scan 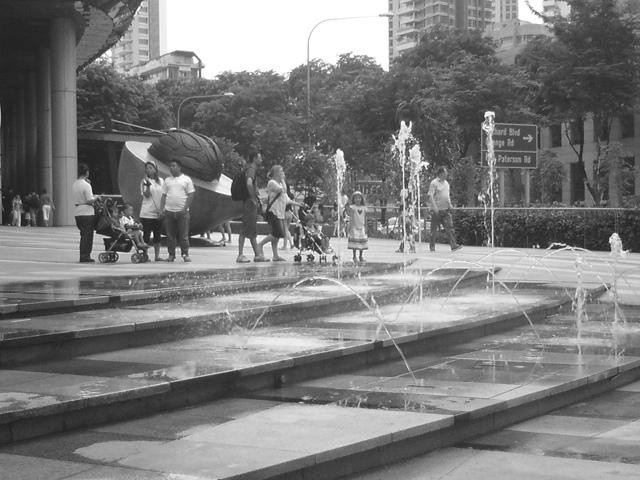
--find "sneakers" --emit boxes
[254,256,270,262]
[183,255,191,262]
[237,255,250,262]
[273,256,286,261]
[168,255,175,261]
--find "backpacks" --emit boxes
[29,193,39,210]
[230,166,252,202]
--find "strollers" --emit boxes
[90,194,151,263]
[286,203,340,264]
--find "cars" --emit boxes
[378,214,429,232]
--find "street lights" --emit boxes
[176,91,236,132]
[305,12,395,157]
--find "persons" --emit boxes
[72,164,102,262]
[139,161,165,261]
[160,159,196,262]
[236,150,270,263]
[428,166,464,252]
[257,165,304,261]
[279,185,338,250]
[200,221,232,243]
[395,189,415,253]
[347,191,369,262]
[2,189,55,227]
[119,203,150,250]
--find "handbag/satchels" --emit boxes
[263,211,275,222]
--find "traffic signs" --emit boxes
[480,122,540,170]
